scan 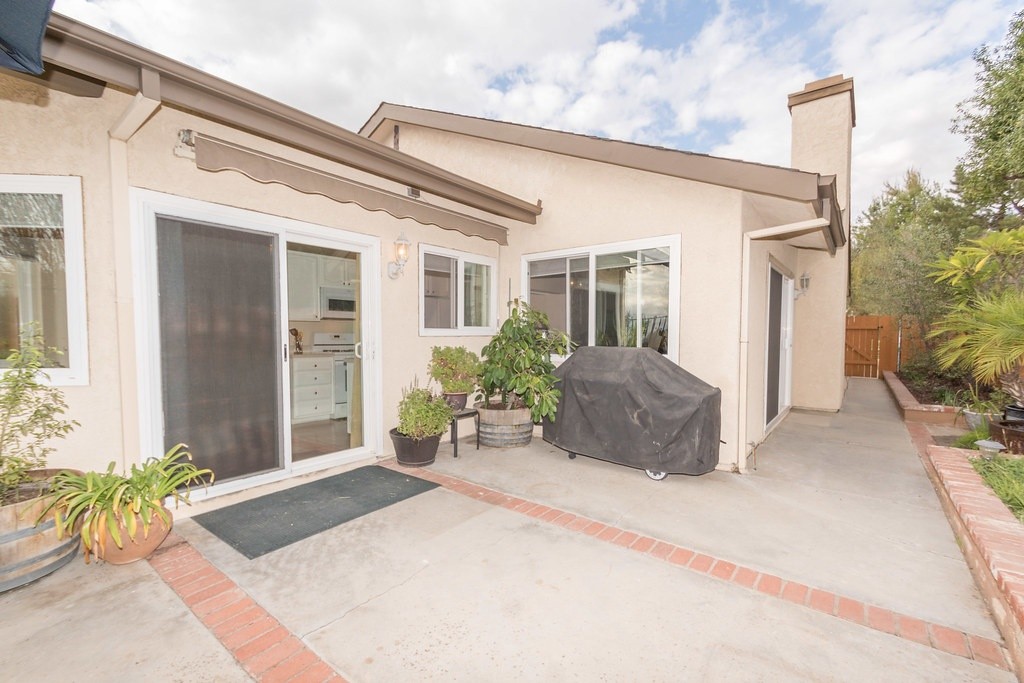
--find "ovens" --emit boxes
[320,286,356,319]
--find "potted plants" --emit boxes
[472,295,580,448]
[924,283,1024,420]
[426,345,482,411]
[15,441,216,566]
[952,377,1005,433]
[0,321,89,594]
[389,373,460,468]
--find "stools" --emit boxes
[437,408,481,458]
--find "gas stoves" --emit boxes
[313,333,355,352]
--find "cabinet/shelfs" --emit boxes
[290,354,335,424]
[319,255,357,290]
[288,251,320,322]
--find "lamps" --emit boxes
[794,270,812,299]
[387,231,412,279]
